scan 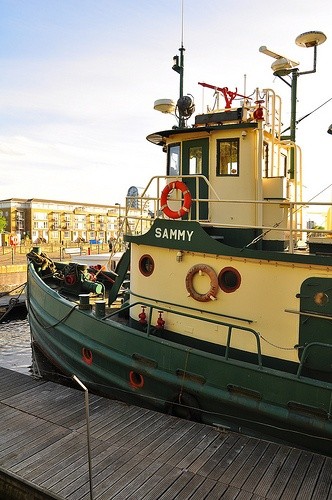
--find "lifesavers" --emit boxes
[185,264,219,303]
[160,181,193,219]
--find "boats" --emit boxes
[23,0,332,459]
